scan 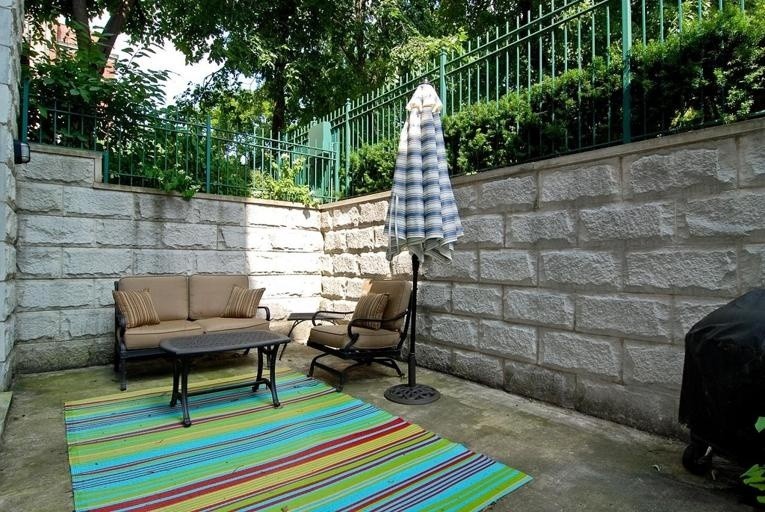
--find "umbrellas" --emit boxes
[378,82,467,354]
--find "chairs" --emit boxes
[306,280,413,393]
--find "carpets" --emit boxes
[63,365,535,512]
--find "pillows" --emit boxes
[351,292,390,331]
[220,284,266,319]
[113,287,161,329]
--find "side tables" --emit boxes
[279,313,346,360]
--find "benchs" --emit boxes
[113,273,270,391]
